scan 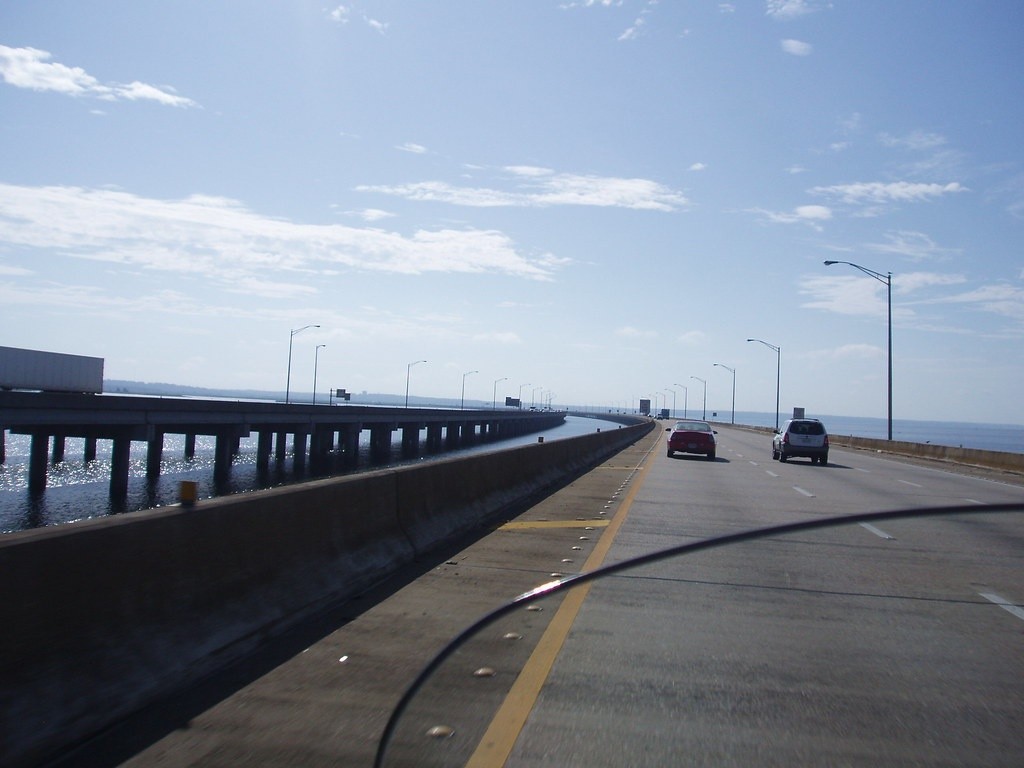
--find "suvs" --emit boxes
[773,418,829,464]
[657,414,663,419]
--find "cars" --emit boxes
[665,420,718,459]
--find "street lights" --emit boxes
[747,339,781,432]
[518,384,531,410]
[674,383,687,419]
[714,363,735,424]
[532,387,554,410]
[286,325,320,405]
[823,260,893,439]
[406,360,427,408]
[494,377,508,410]
[462,371,478,409]
[691,376,706,420]
[664,389,676,418]
[314,345,326,404]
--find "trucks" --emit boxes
[662,409,670,419]
